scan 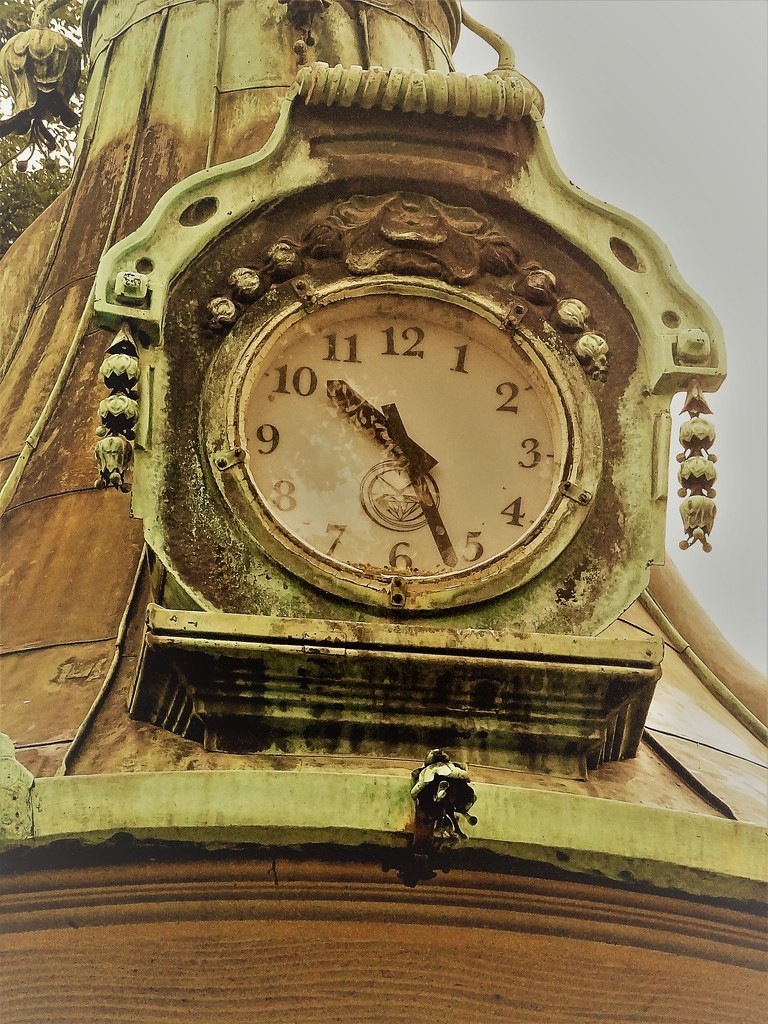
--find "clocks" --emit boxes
[228,274,582,594]
